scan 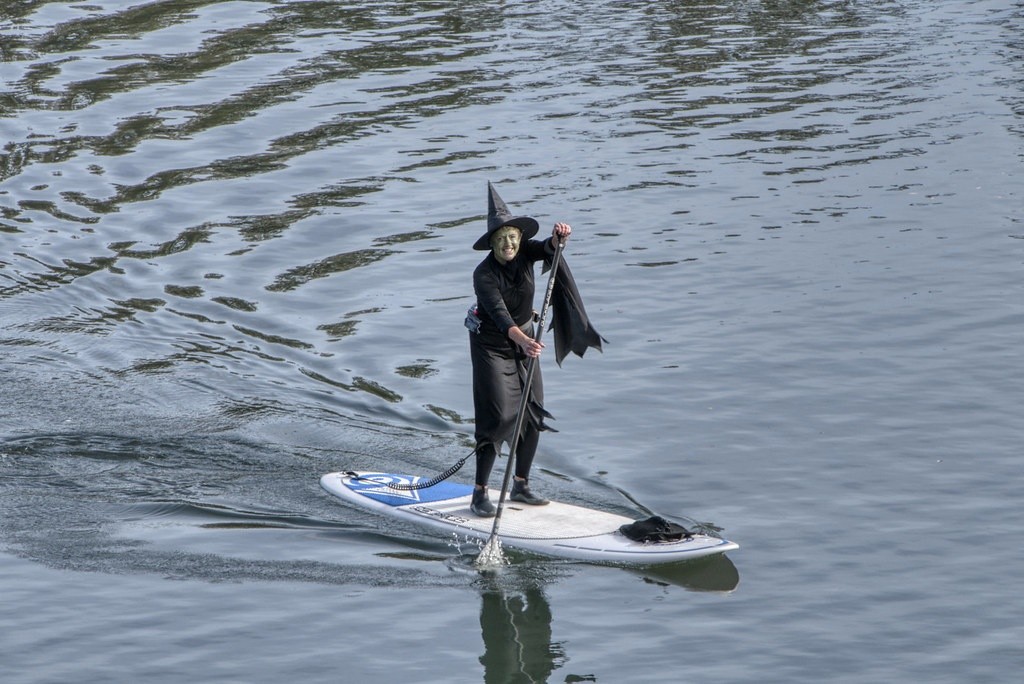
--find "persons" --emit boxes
[469,179,572,518]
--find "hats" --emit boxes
[473,180,539,250]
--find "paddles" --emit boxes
[475,229,569,565]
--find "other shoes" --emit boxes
[510,475,550,505]
[470,485,497,517]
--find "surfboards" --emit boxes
[319,469,741,567]
